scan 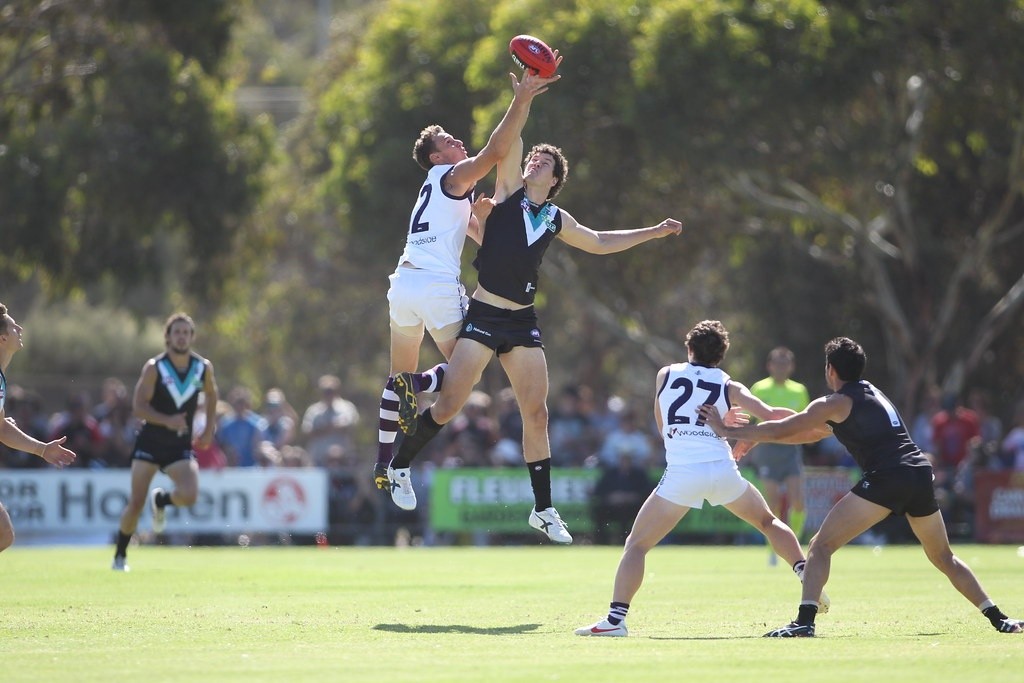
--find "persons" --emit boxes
[387,49,684,544]
[576,319,832,636]
[695,337,1023,636]
[740,348,811,564]
[111,313,219,572]
[374,69,549,495]
[0,376,1024,542]
[0,303,76,551]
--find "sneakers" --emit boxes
[392,372,417,436]
[762,622,815,638]
[373,463,391,496]
[387,457,417,510]
[795,562,831,613]
[113,555,128,571]
[996,616,1024,633]
[528,505,574,545]
[574,617,628,637]
[150,486,167,533]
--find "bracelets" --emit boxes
[41,444,47,458]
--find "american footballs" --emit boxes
[508,33,558,79]
